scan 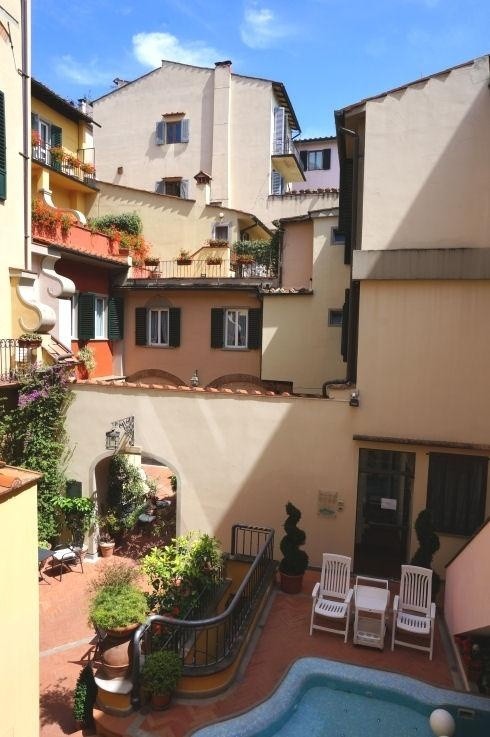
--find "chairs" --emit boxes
[391,564,436,660]
[309,553,353,643]
[53,538,84,582]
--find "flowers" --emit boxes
[141,528,227,651]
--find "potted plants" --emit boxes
[277,502,309,593]
[139,651,184,711]
[18,333,42,349]
[409,508,440,602]
[97,532,115,557]
[75,345,96,380]
[118,239,130,255]
[143,238,257,266]
[86,560,151,637]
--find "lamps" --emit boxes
[189,370,199,387]
[349,389,359,407]
[105,426,120,450]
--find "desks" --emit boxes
[352,576,390,652]
[38,546,57,585]
